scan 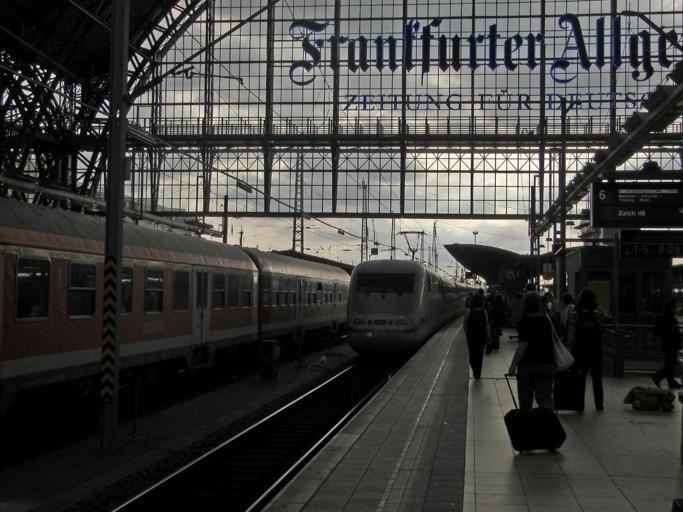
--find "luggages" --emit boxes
[552,362,587,415]
[501,368,569,451]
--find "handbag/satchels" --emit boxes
[622,384,677,412]
[544,309,578,370]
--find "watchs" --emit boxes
[508,367,515,373]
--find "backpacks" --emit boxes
[569,323,603,368]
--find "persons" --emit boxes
[565,287,615,414]
[507,290,558,455]
[462,293,491,379]
[464,283,575,356]
[650,293,683,389]
